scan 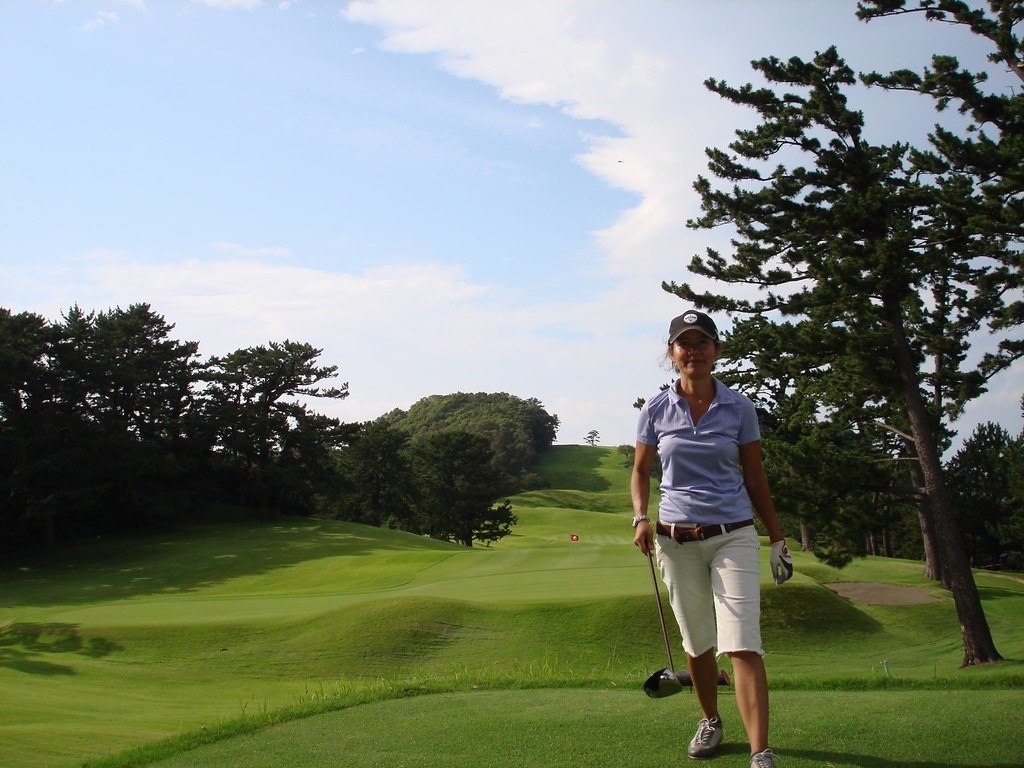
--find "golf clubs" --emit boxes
[643,544,683,698]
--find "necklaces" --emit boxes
[677,389,711,405]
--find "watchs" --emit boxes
[631,514,652,528]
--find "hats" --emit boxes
[668,309,719,347]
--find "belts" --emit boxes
[656,517,755,544]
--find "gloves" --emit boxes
[768,541,794,586]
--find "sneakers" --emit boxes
[688,716,723,760]
[750,748,784,768]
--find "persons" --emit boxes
[631,308,793,768]
